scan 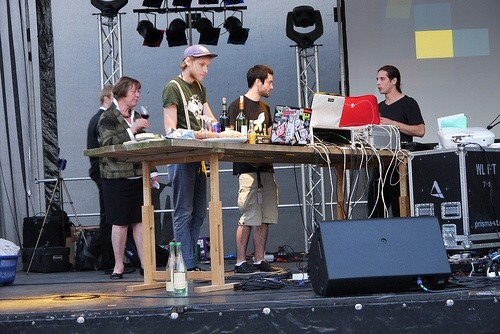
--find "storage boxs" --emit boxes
[408,144,500,251]
[22,247,70,274]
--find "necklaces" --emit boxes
[123,115,131,118]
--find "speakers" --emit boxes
[307,216,451,297]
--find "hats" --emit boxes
[184,44,218,58]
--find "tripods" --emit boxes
[25,166,97,275]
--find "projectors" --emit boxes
[438,127,495,150]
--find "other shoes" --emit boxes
[188,267,206,272]
[105,267,135,274]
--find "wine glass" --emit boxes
[140,105,150,130]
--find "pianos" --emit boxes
[400,141,439,152]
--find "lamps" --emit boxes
[286,5,323,48]
[165,11,188,48]
[136,12,165,47]
[195,10,221,45]
[224,10,249,44]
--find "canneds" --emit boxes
[211,122,221,133]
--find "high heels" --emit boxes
[139,268,144,277]
[111,270,124,279]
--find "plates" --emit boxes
[203,137,245,144]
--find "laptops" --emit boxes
[270,105,313,146]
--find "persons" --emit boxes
[87,85,145,275]
[96,76,158,280]
[360,63,426,222]
[224,63,289,274]
[162,45,218,275]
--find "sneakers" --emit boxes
[234,262,257,275]
[252,260,277,271]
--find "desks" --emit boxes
[84,138,409,294]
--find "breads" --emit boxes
[193,130,244,139]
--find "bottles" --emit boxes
[220,96,230,132]
[235,95,248,138]
[248,120,256,144]
[173,242,188,296]
[165,241,175,297]
